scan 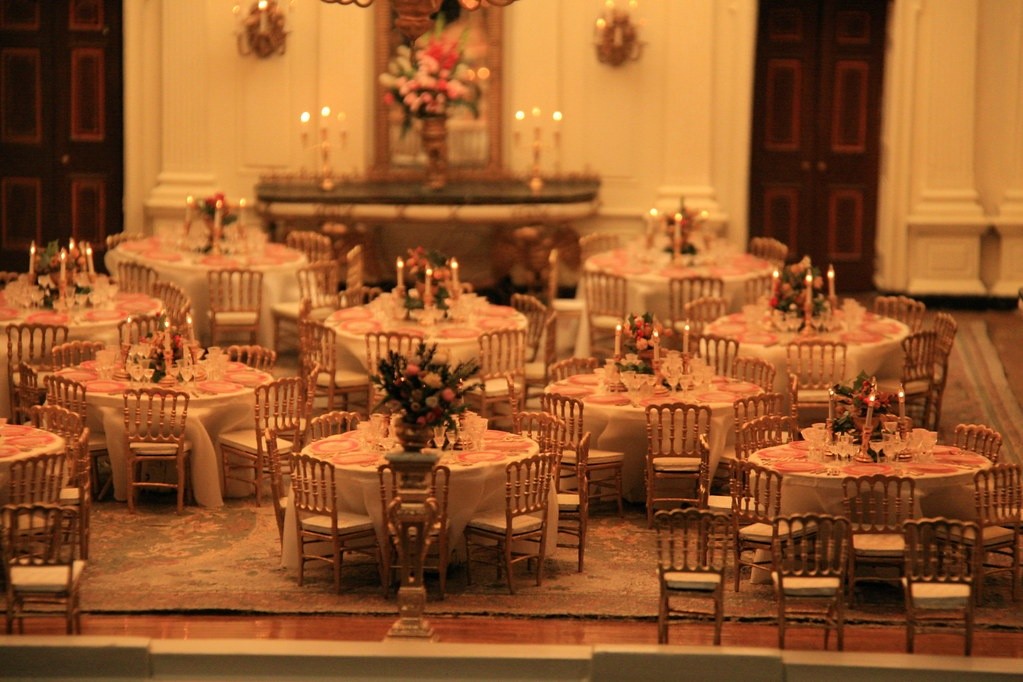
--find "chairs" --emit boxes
[0,231,1023,655]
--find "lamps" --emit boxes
[230,0,290,58]
[593,1,646,67]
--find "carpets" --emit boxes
[0,319,1023,628]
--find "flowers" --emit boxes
[189,192,238,224]
[402,246,463,310]
[379,11,482,140]
[616,313,655,375]
[35,239,91,309]
[368,340,483,449]
[771,265,845,317]
[137,328,183,383]
[666,207,699,236]
[832,368,906,437]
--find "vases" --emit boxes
[422,116,448,189]
[395,417,432,452]
[205,219,225,235]
[639,347,662,362]
[856,416,879,431]
[50,271,73,286]
[415,280,438,295]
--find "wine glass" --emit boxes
[801,422,938,463]
[742,299,867,340]
[371,292,489,332]
[94,345,224,387]
[358,412,488,452]
[4,274,119,314]
[593,354,714,403]
[154,223,269,262]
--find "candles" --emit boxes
[29,197,906,427]
[299,106,563,149]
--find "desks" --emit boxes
[0,424,66,510]
[544,370,767,505]
[40,359,276,509]
[105,237,321,351]
[700,308,910,421]
[749,441,995,584]
[575,249,775,360]
[321,302,528,390]
[282,429,559,563]
[1,281,167,417]
[256,179,601,221]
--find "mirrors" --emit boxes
[372,0,502,181]
